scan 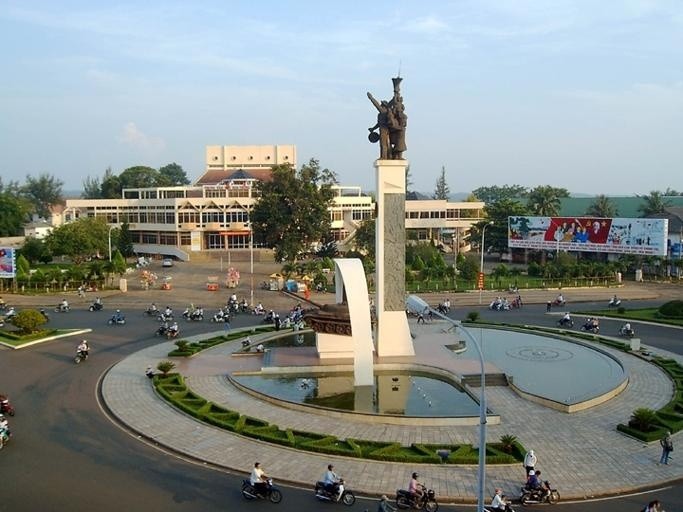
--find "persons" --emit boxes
[316,280,324,292]
[546,301,552,313]
[323,465,345,504]
[389,92,407,160]
[555,294,563,305]
[377,495,396,512]
[112,309,125,323]
[611,295,617,305]
[406,298,450,324]
[5,306,15,315]
[553,220,599,243]
[249,462,268,500]
[485,294,521,312]
[643,501,660,512]
[588,317,598,328]
[365,91,391,160]
[77,339,88,357]
[522,449,537,476]
[0,412,11,432]
[92,296,102,309]
[143,291,304,352]
[557,312,571,326]
[658,431,672,465]
[60,298,68,311]
[489,489,507,510]
[624,320,631,335]
[526,470,548,501]
[407,471,423,509]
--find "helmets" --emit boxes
[495,489,501,494]
[328,464,334,470]
[413,473,418,478]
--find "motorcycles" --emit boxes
[581,318,601,334]
[75,345,92,364]
[315,286,329,296]
[552,299,568,309]
[142,297,304,354]
[393,482,439,510]
[0,393,15,451]
[239,475,283,503]
[616,324,635,337]
[315,477,357,506]
[606,298,622,308]
[0,293,128,330]
[520,481,560,507]
[403,302,453,320]
[487,297,526,312]
[556,316,575,328]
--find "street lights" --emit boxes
[477,220,495,305]
[246,222,255,308]
[108,224,119,263]
[402,292,487,512]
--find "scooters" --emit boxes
[488,502,514,512]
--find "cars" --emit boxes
[162,258,173,267]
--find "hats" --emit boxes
[529,450,534,455]
[529,470,535,476]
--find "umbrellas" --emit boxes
[270,271,283,290]
[300,272,315,288]
